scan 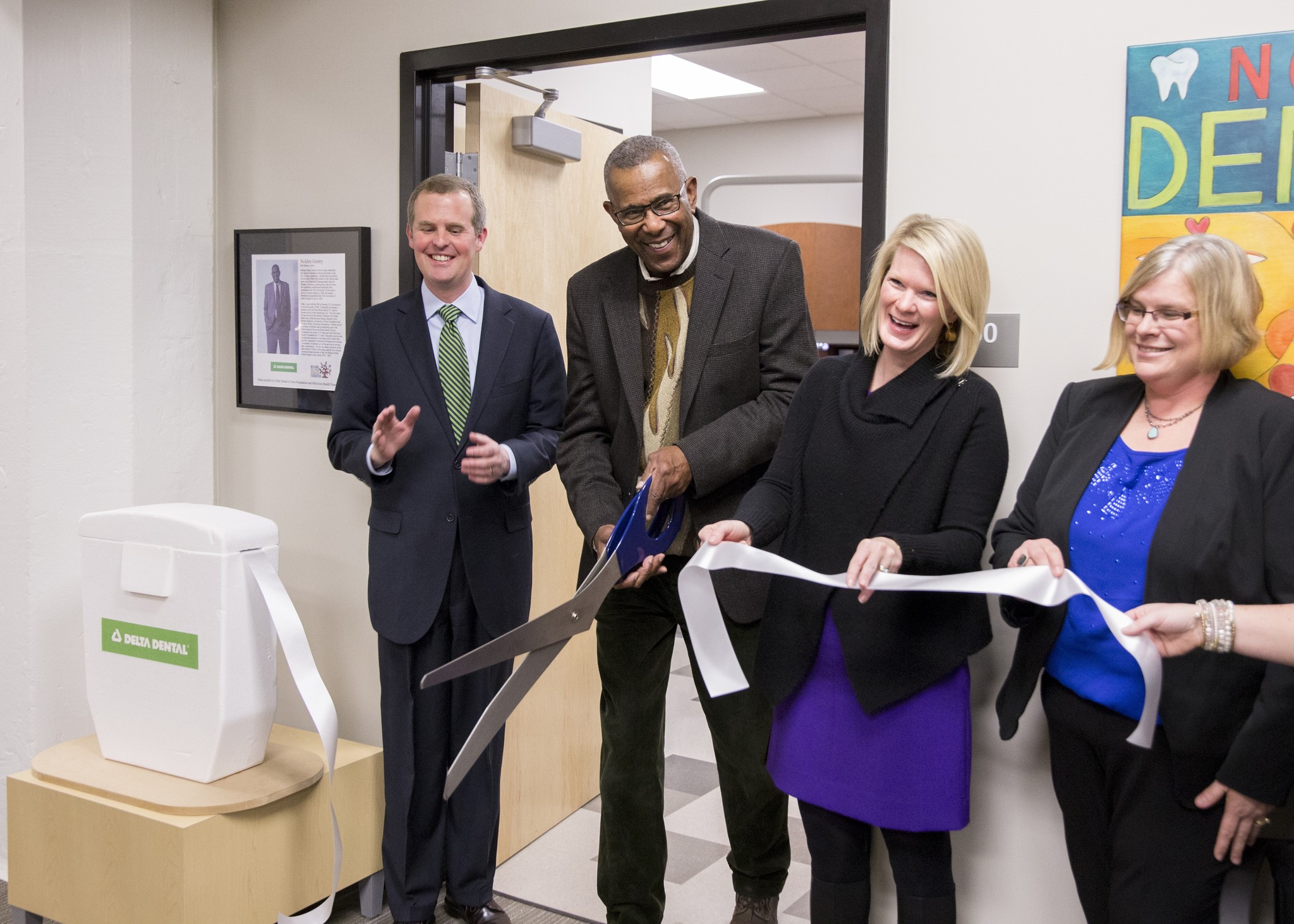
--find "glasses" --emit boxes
[1115,299,1198,327]
[610,178,686,226]
[272,271,279,274]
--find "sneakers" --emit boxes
[729,894,780,924]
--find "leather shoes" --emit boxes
[445,898,511,924]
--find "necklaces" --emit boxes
[1144,404,1202,442]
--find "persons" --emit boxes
[988,235,1294,924]
[1119,602,1294,668]
[698,217,1010,923]
[327,172,567,924]
[563,136,821,924]
[264,265,292,354]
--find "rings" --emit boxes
[491,467,494,476]
[877,564,890,574]
[1017,554,1031,567]
[1254,817,1271,828]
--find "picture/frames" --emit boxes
[233,225,372,414]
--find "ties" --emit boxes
[275,284,281,326]
[437,303,471,450]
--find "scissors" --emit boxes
[421,476,687,800]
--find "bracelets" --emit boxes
[1195,599,1234,654]
